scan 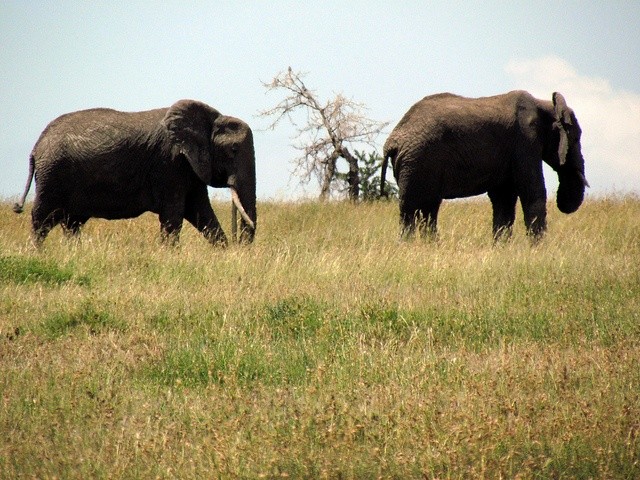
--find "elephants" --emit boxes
[11,99,257,252]
[380,91,591,251]
